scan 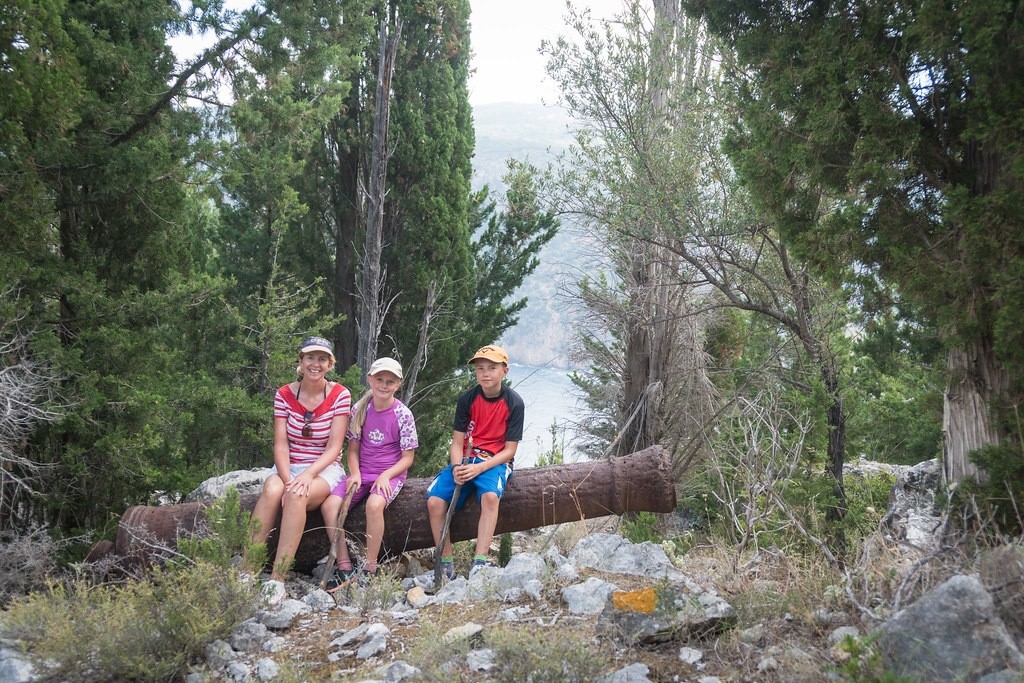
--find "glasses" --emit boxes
[302,411,315,438]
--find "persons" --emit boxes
[235,336,352,607]
[320,357,420,593]
[425,345,526,594]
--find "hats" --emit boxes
[369,357,402,379]
[468,346,508,367]
[302,336,334,356]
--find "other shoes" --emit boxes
[325,570,356,592]
[466,559,485,579]
[427,562,457,594]
[358,569,374,589]
